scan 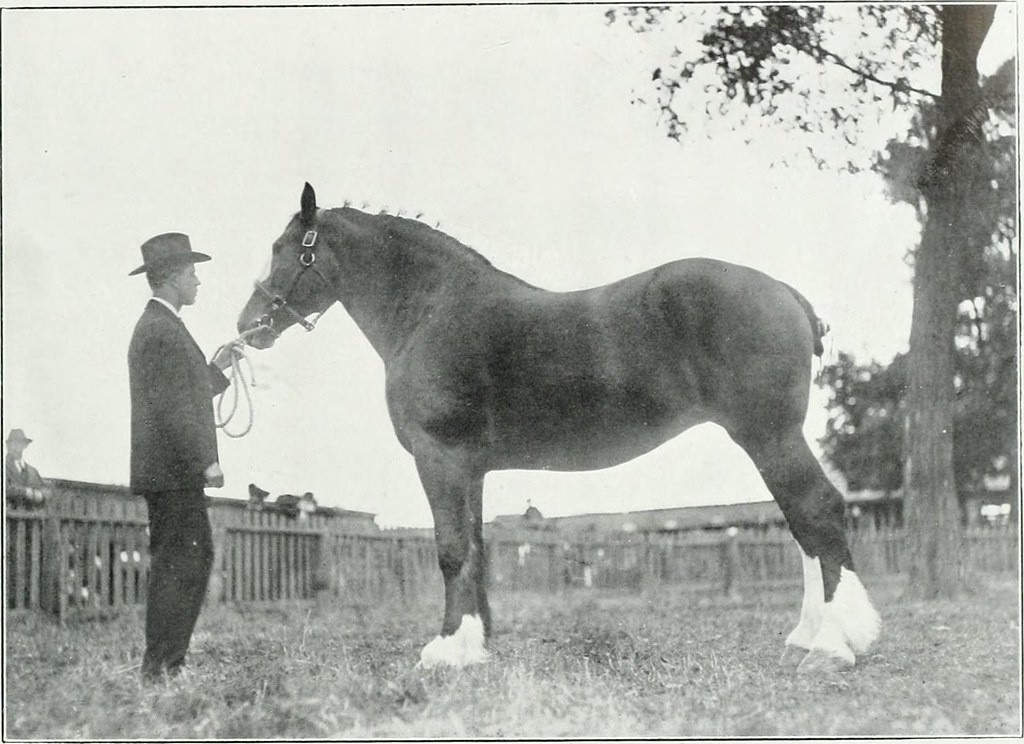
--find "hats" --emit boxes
[129,233,211,276]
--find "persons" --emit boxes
[4,428,50,607]
[128,232,245,689]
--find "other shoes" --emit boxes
[143,667,187,718]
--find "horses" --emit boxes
[236,179,883,677]
